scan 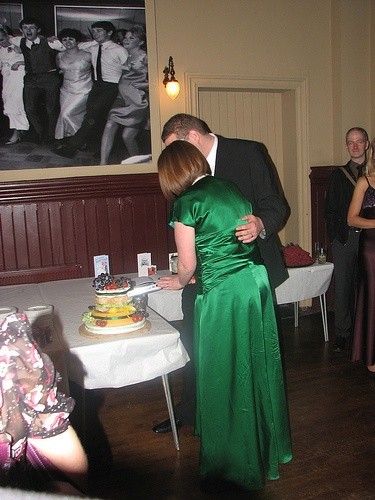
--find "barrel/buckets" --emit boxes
[0,306,16,326]
[23,305,52,324]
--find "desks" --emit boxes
[0,258,335,451]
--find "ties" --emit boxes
[356,165,365,178]
[31,42,35,50]
[96,45,103,85]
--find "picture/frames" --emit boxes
[0,0,160,173]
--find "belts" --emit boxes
[349,227,362,233]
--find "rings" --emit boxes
[167,284,168,287]
[251,233,253,237]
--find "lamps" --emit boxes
[161,57,180,100]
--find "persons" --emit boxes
[0,19,149,165]
[326,127,370,352]
[153,113,291,431]
[347,137,375,371]
[0,314,89,476]
[157,140,293,500]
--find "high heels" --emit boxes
[5,134,22,145]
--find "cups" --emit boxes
[318,247,327,263]
[132,294,147,315]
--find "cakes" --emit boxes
[82,273,143,327]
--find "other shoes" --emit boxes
[51,147,75,159]
[152,415,184,433]
[366,366,375,379]
[332,344,345,352]
